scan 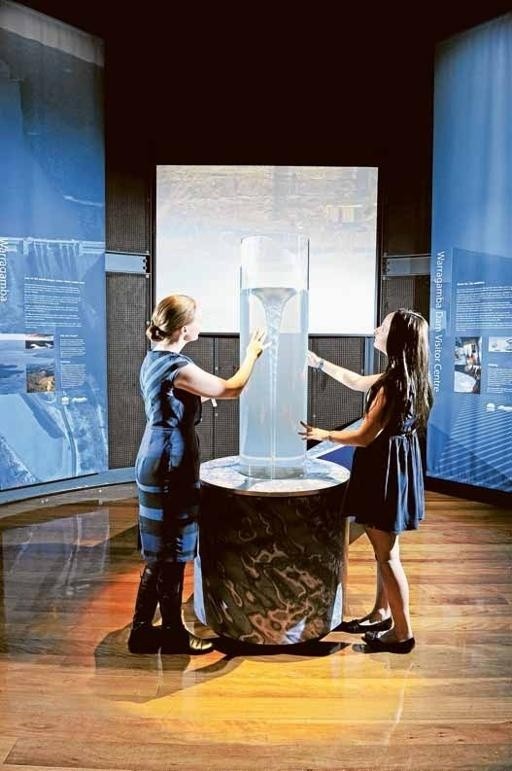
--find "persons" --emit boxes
[128,293,272,654]
[295,307,435,658]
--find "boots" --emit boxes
[128,565,162,653]
[157,563,214,654]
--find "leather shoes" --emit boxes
[345,617,392,633]
[361,632,415,653]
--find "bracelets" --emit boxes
[318,357,325,368]
[327,429,334,443]
